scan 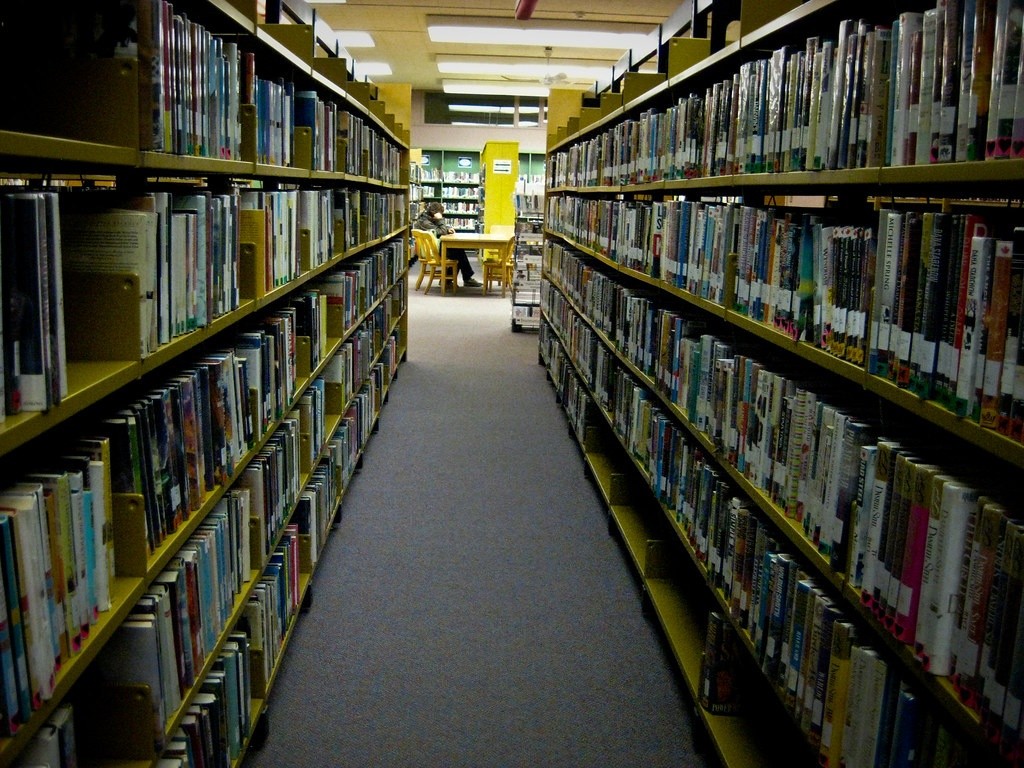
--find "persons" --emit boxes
[416,202,482,290]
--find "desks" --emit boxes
[439,233,511,299]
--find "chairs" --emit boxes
[418,231,459,297]
[482,235,515,299]
[411,228,448,291]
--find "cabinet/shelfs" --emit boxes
[520,0,1024,768]
[0,0,518,768]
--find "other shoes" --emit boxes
[439,281,459,289]
[464,278,481,287]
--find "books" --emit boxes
[512,174,545,327]
[539,0,1024,768]
[409,162,486,259]
[0,237,406,768]
[151,0,401,184]
[0,188,404,425]
